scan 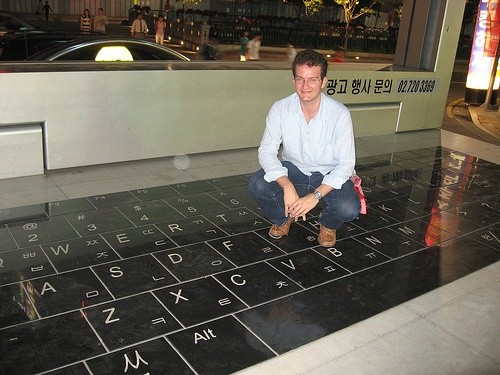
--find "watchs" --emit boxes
[311,189,323,202]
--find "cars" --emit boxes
[23,34,191,63]
[0,13,77,61]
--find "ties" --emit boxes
[139,19,142,31]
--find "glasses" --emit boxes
[295,77,321,84]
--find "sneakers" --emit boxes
[317,223,336,247]
[268,219,293,239]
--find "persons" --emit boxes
[80,9,93,32]
[154,15,166,45]
[92,8,109,33]
[285,41,298,63]
[238,29,261,62]
[248,49,362,247]
[235,251,355,359]
[130,13,149,40]
[35,0,53,25]
